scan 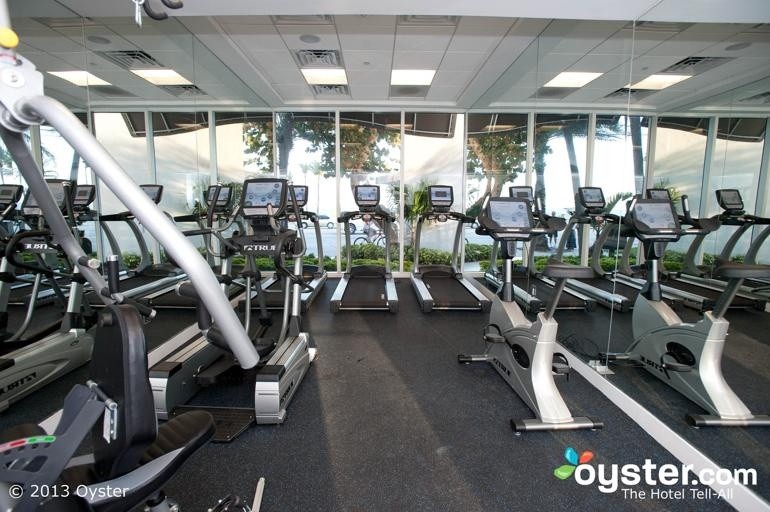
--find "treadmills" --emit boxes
[0,184,766,313]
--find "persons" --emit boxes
[565,226,577,253]
[547,211,559,246]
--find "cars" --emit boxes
[300,213,335,229]
[340,214,369,235]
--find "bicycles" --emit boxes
[352,229,387,249]
